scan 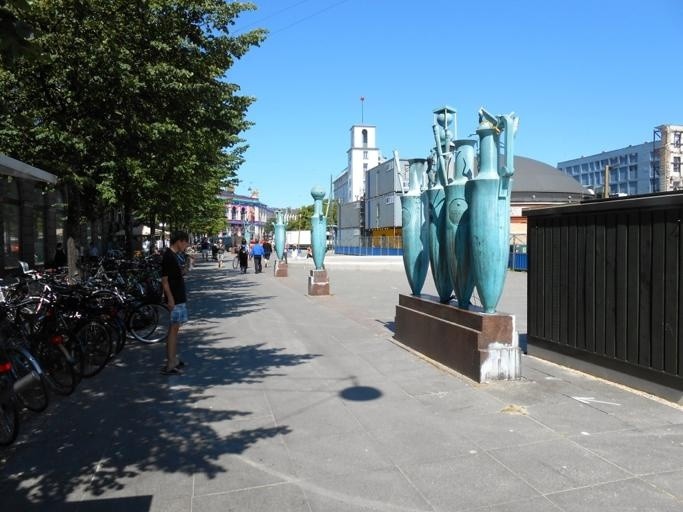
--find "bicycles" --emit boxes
[0,248,169,436]
[231,255,239,269]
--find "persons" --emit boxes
[193,236,298,274]
[159,229,191,374]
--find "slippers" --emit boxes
[175,361,188,369]
[160,369,183,375]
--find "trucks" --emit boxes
[285,230,334,250]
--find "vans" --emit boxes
[201,237,246,252]
